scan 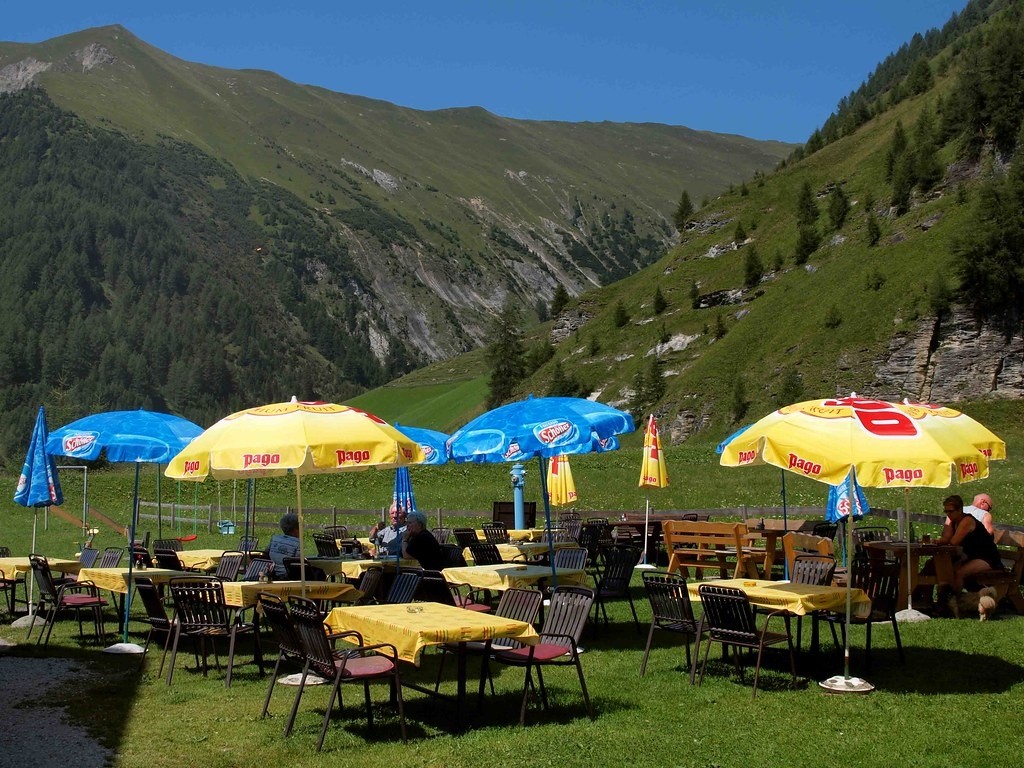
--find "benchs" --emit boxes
[580,520,663,562]
[618,511,711,548]
[660,518,760,580]
[746,518,828,577]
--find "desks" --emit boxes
[0,557,80,614]
[674,578,871,674]
[333,535,381,556]
[861,537,961,616]
[306,557,421,581]
[474,529,565,542]
[202,581,364,662]
[151,548,264,583]
[78,566,205,633]
[440,561,587,628]
[462,541,581,566]
[321,601,540,736]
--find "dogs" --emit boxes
[942,584,996,621]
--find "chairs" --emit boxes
[0,509,906,753]
[968,530,1024,618]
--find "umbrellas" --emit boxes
[634,412,671,569]
[714,390,1024,693]
[446,394,636,655]
[44,406,206,653]
[11,404,65,627]
[164,395,451,607]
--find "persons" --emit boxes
[944,493,994,536]
[369,503,407,593]
[400,511,439,569]
[934,495,1002,618]
[262,513,301,581]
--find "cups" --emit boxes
[923,535,930,544]
[379,547,389,558]
[340,547,346,557]
[362,544,368,552]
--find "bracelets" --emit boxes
[403,541,407,542]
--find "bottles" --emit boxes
[136,554,143,569]
[353,535,359,552]
[374,538,380,553]
[909,522,915,543]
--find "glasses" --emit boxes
[985,501,992,511]
[942,508,958,513]
